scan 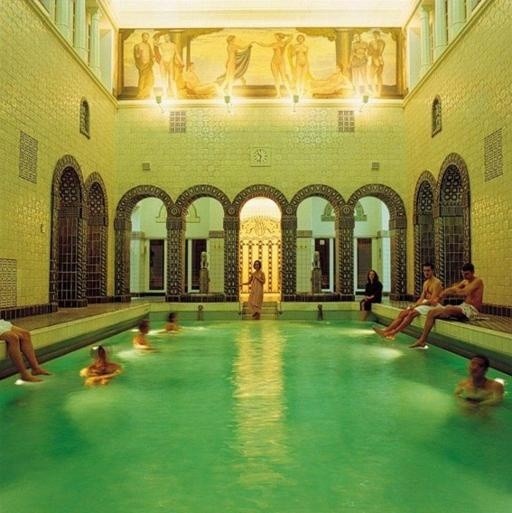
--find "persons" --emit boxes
[235,260,265,320]
[451,354,503,418]
[357,271,384,321]
[132,318,159,352]
[78,345,122,385]
[164,312,183,334]
[133,30,385,100]
[372,263,445,339]
[408,261,485,349]
[0,317,54,384]
